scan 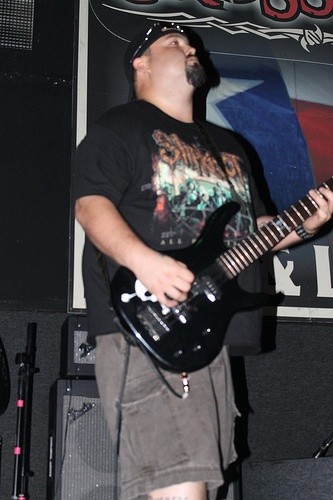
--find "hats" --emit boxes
[126,21,188,67]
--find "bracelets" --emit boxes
[295,225,314,240]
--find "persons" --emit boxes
[72,22,333,500]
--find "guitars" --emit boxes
[111,178,333,374]
